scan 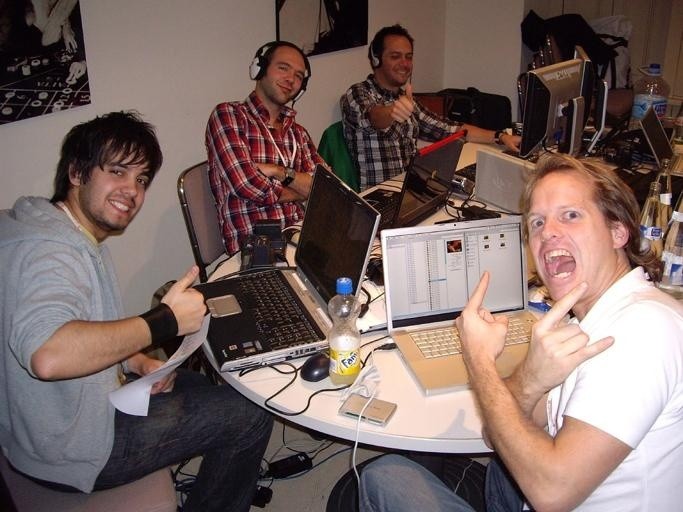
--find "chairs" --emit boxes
[0,450,274,511]
[318,117,360,193]
[175,160,227,282]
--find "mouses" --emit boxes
[300,353,329,381]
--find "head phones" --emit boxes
[369,41,382,69]
[248,38,312,102]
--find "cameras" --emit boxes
[238,219,288,275]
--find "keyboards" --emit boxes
[455,144,520,181]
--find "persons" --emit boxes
[1,107,277,512]
[339,20,522,192]
[201,42,335,255]
[357,150,683,512]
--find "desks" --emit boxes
[194,134,682,512]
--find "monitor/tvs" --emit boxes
[573,46,609,155]
[519,59,585,160]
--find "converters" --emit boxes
[268,452,313,478]
[250,485,272,508]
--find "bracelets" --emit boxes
[493,129,508,146]
[137,302,180,349]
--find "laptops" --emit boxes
[191,163,381,374]
[361,130,468,238]
[639,106,683,177]
[380,215,539,397]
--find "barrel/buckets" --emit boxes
[627,62,670,132]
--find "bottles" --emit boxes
[655,160,672,235]
[328,278,362,387]
[654,191,683,299]
[637,180,663,262]
[627,63,671,143]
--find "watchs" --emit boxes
[279,166,297,188]
